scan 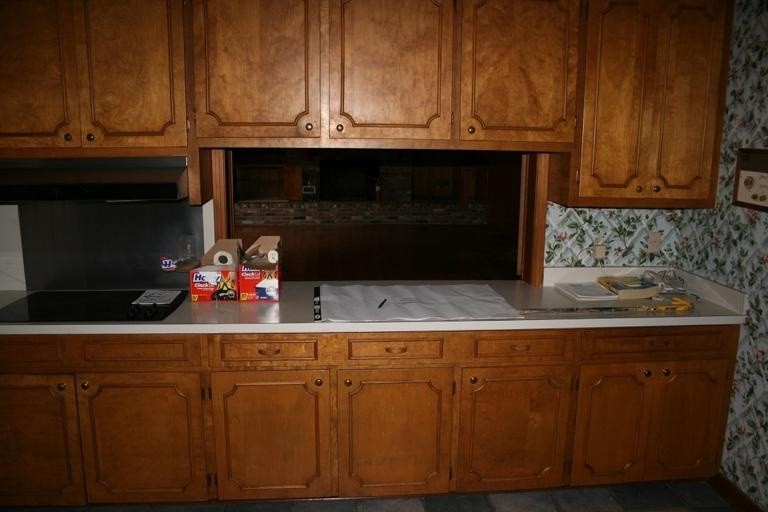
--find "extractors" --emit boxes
[0,160,188,205]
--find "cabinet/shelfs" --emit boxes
[195,1,459,150]
[0,1,195,160]
[211,332,454,511]
[454,332,575,491]
[547,2,734,210]
[1,333,211,509]
[459,2,583,152]
[564,327,742,488]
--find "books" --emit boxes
[555,276,658,302]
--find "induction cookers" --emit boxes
[0,286,183,325]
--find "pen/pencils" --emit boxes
[378,299,386,308]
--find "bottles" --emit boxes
[251,249,278,270]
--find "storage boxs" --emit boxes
[189,233,282,304]
